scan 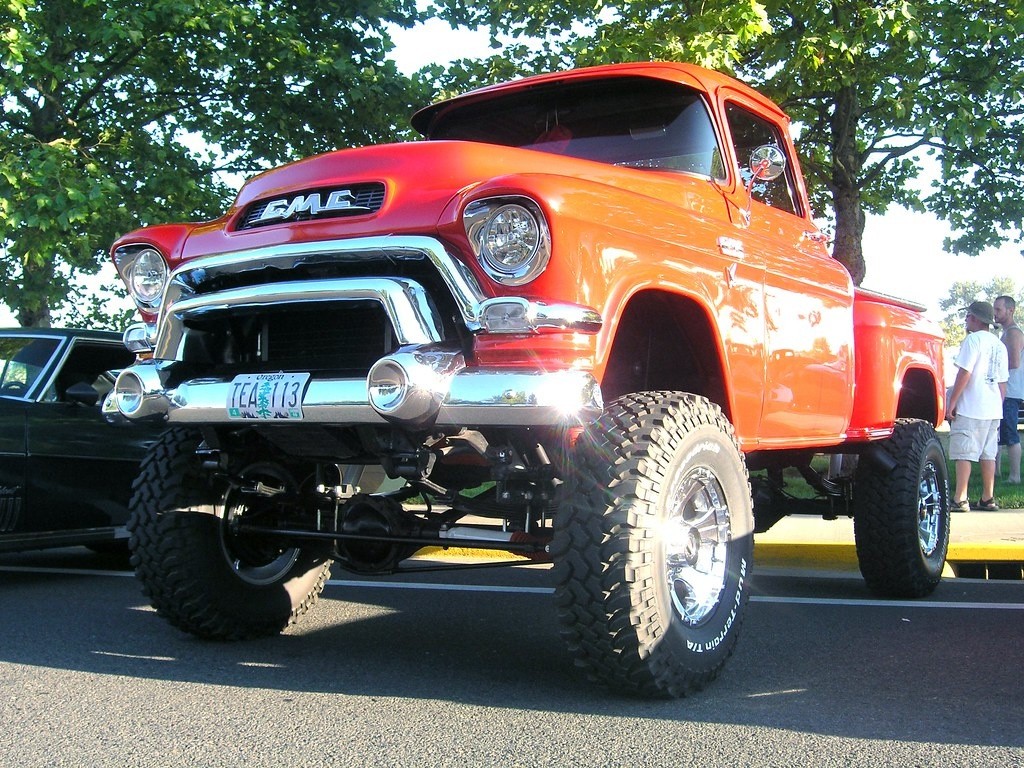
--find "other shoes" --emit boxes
[998,478,1022,487]
[995,475,1002,481]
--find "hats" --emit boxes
[958,302,994,325]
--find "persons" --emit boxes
[943,301,1010,511]
[993,296,1024,485]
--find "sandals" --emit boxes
[971,497,998,511]
[950,500,970,512]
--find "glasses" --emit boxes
[967,312,972,318]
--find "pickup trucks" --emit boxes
[101,61,953,699]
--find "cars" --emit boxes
[0,328,164,567]
